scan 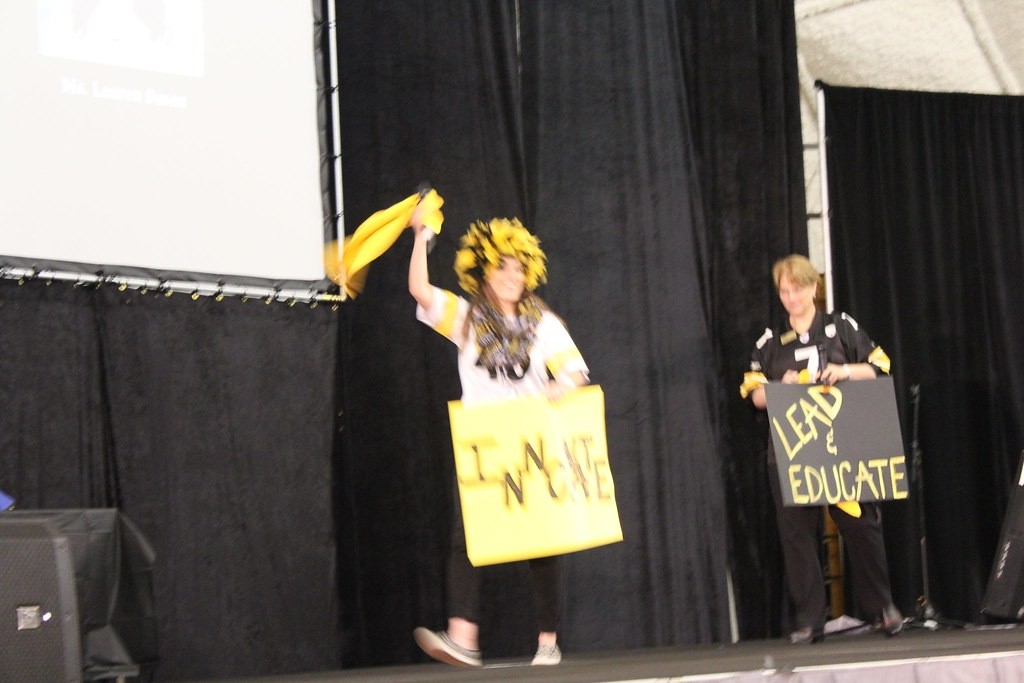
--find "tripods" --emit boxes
[895,385,975,632]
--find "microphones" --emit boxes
[817,344,830,393]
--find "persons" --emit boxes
[741,253,903,642]
[406,174,591,668]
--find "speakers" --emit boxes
[0,512,87,683]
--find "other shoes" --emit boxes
[789,627,818,642]
[881,604,904,638]
[413,628,482,669]
[530,644,561,665]
